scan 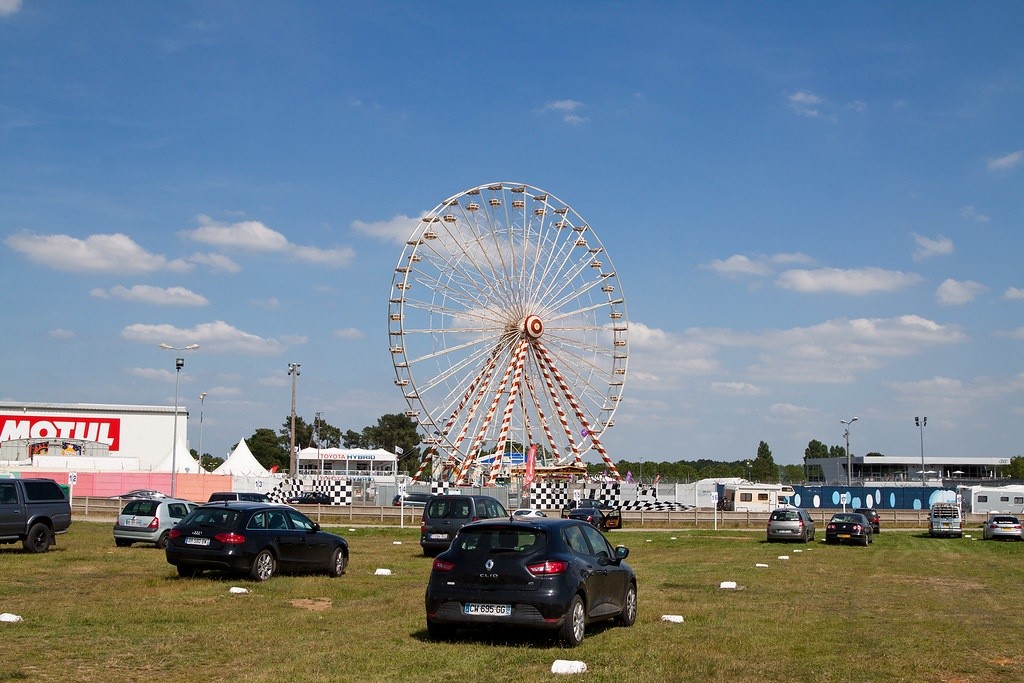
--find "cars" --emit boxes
[108,489,185,501]
[983,515,1024,542]
[561,499,623,532]
[512,509,548,519]
[767,507,815,543]
[114,500,212,550]
[825,514,873,547]
[166,503,350,582]
[287,492,331,505]
[425,519,637,649]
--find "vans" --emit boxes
[420,494,509,557]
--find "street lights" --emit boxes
[915,417,927,485]
[199,393,206,473]
[840,417,858,487]
[159,343,200,500]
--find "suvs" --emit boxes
[209,491,272,515]
[851,508,881,534]
[392,492,445,508]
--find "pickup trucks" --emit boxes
[927,503,963,538]
[0,477,72,554]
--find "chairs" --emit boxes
[175,507,182,517]
[212,514,224,523]
[446,506,464,517]
[269,515,283,529]
[499,532,518,548]
